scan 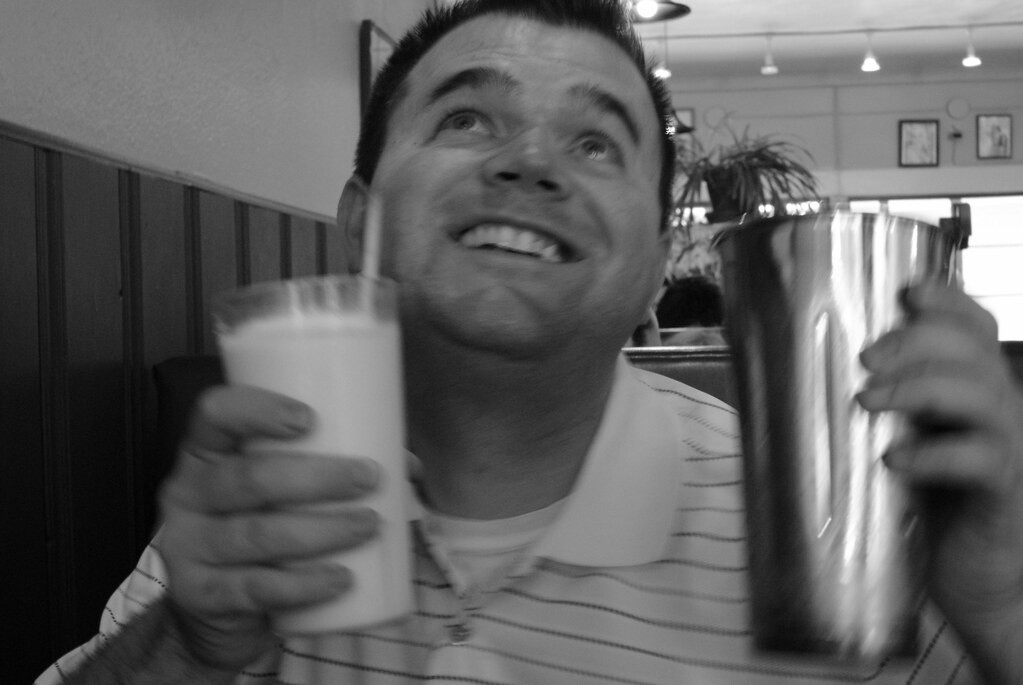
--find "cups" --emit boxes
[210,275,418,639]
[716,203,971,665]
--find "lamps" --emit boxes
[761,35,779,73]
[963,27,983,67]
[628,0,690,23]
[651,22,672,78]
[861,32,880,71]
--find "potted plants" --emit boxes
[670,108,821,224]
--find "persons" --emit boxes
[656,278,725,328]
[31,1,1023,685]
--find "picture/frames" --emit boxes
[976,112,1013,160]
[899,118,939,167]
